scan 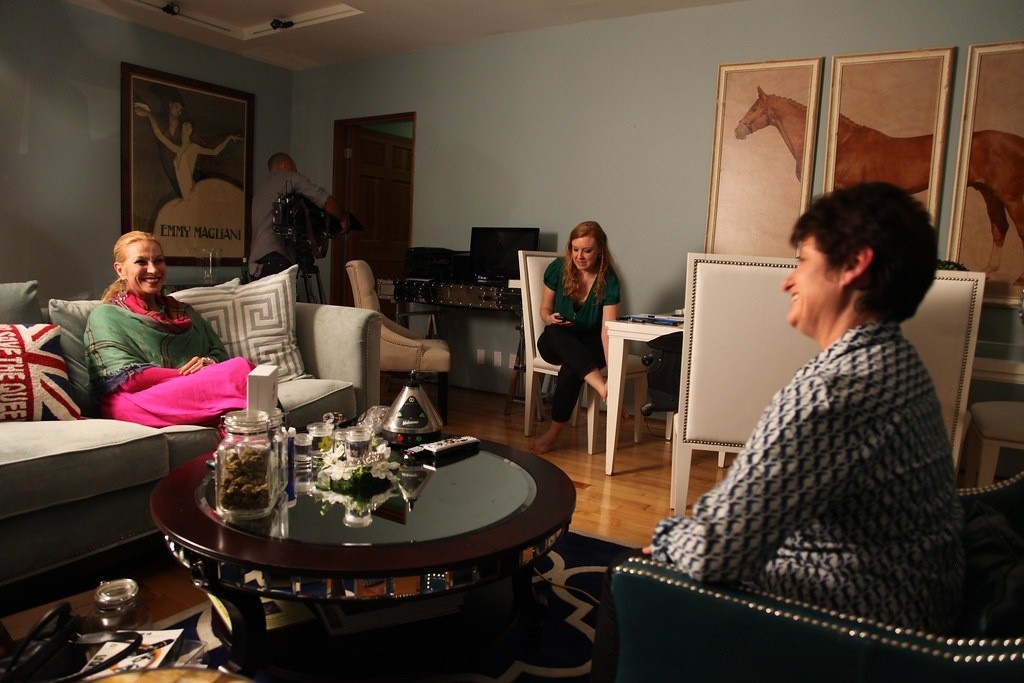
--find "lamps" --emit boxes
[271,19,294,29]
[161,4,180,15]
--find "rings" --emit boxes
[202,362,207,366]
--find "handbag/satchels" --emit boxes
[271,180,331,259]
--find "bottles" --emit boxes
[215,409,371,518]
[241,258,247,281]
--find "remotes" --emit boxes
[405,436,483,458]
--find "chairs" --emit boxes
[346,259,451,381]
[517,246,1024,683]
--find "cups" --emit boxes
[195,248,222,283]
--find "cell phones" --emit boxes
[556,316,570,323]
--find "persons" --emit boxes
[248,153,349,281]
[530,221,629,454]
[591,180,969,683]
[84,231,287,440]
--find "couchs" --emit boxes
[0,302,382,585]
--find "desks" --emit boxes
[378,277,544,421]
[602,319,684,476]
[150,431,575,683]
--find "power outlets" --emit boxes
[493,351,502,366]
[509,353,517,369]
[477,349,485,364]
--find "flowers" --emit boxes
[320,436,400,481]
[312,458,402,515]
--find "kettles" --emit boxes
[382,370,443,445]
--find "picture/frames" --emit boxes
[706,41,1024,308]
[116,62,254,268]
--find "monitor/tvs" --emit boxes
[470,227,540,281]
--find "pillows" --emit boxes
[0,264,314,422]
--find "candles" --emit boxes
[344,427,373,459]
[342,502,372,528]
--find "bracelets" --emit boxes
[207,357,215,364]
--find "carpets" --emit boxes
[145,526,649,683]
